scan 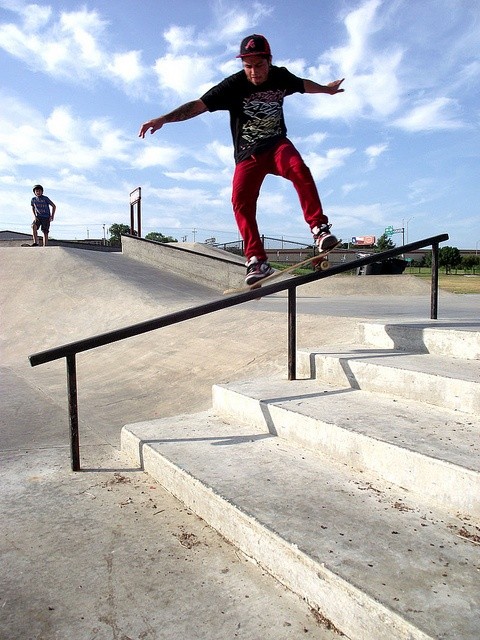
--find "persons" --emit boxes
[139,33,345,285]
[31,185,56,246]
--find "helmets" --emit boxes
[32,184,43,197]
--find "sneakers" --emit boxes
[244,255,276,284]
[312,223,338,252]
[31,243,38,246]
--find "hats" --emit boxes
[235,34,272,59]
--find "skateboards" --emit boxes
[223,239,343,301]
